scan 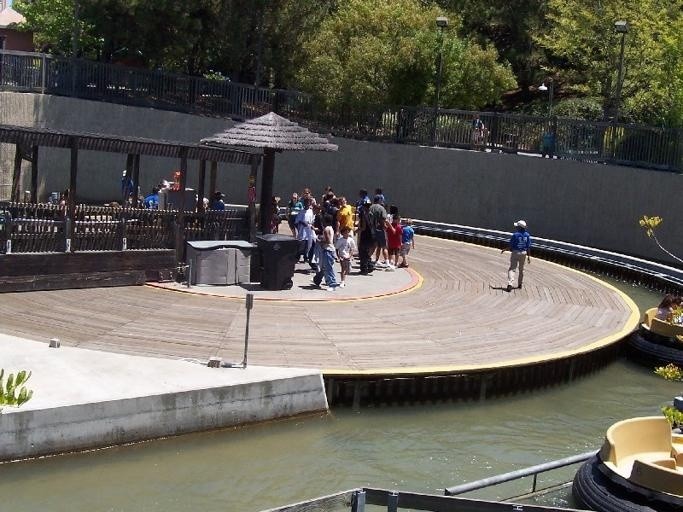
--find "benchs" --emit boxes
[644,306,682,340]
[600,414,683,497]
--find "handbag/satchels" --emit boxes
[367,226,384,248]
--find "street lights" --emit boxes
[610,20,628,160]
[429,17,448,147]
[539,77,552,137]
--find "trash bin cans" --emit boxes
[256,233,299,291]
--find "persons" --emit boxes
[109,170,225,225]
[468,112,485,152]
[541,126,555,160]
[270,185,415,293]
[655,294,683,325]
[498,218,531,293]
[57,194,65,205]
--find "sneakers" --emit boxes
[375,260,409,272]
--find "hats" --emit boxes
[514,220,526,229]
[507,284,522,292]
